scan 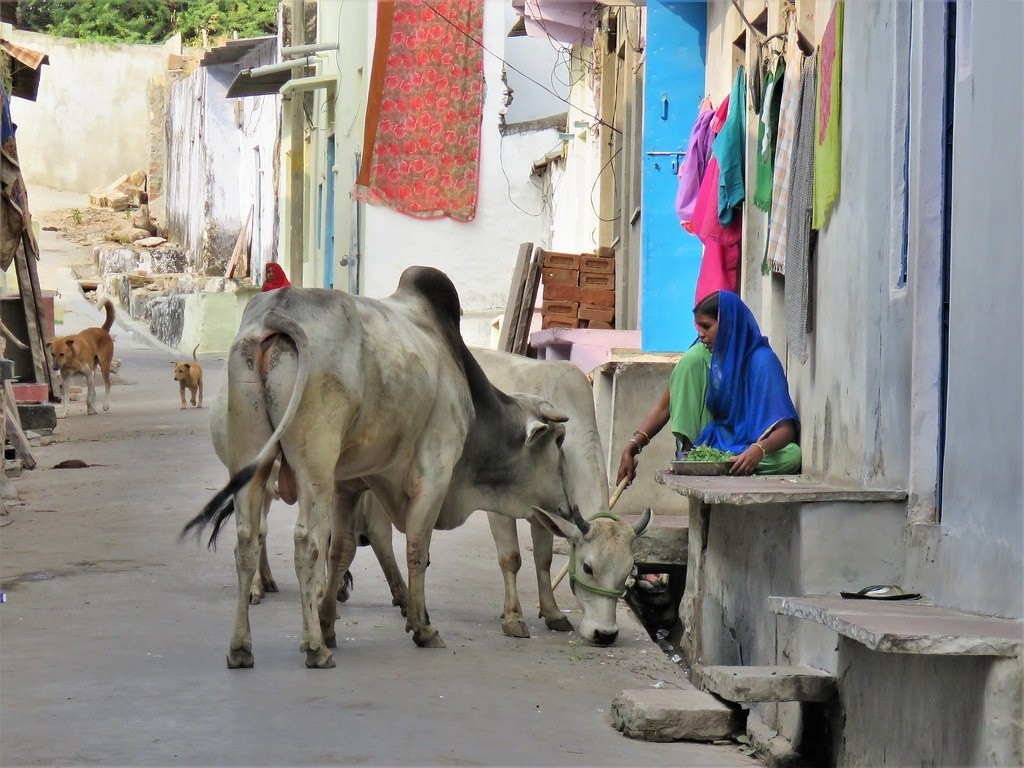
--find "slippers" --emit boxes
[840,584,921,600]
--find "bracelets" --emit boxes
[629,439,641,453]
[633,431,650,446]
[750,443,765,458]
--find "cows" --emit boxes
[176,265,651,672]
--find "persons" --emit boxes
[261,263,291,292]
[617,290,803,489]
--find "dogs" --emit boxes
[170,343,204,410]
[46,296,115,419]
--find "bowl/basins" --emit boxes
[666,459,736,475]
